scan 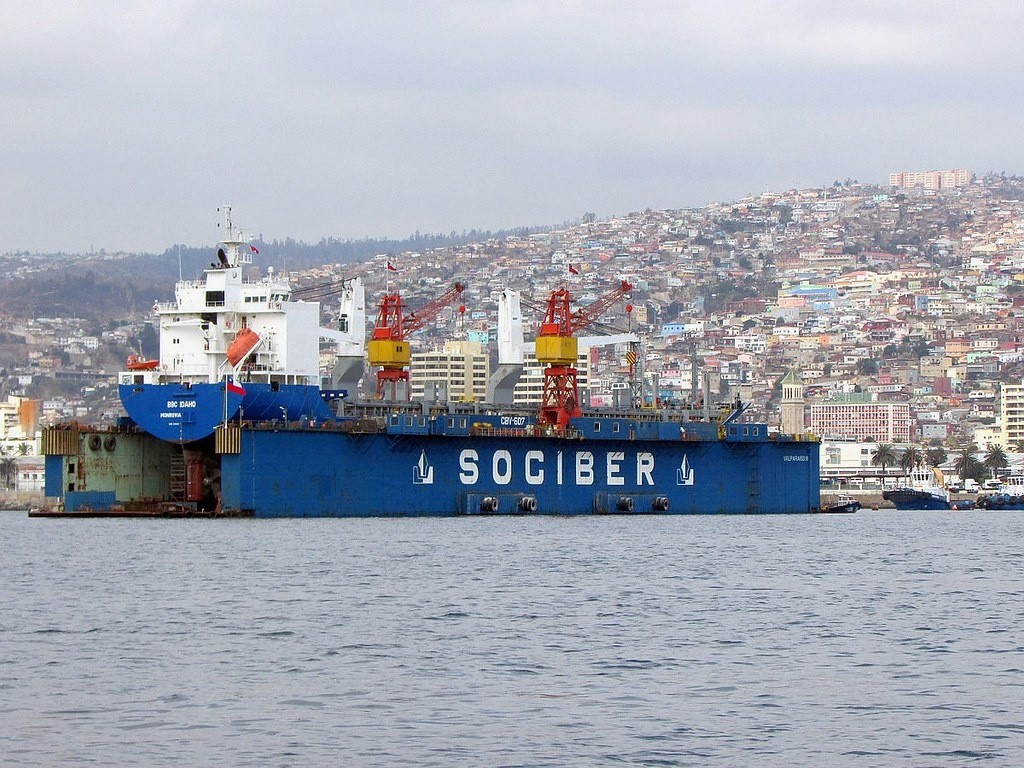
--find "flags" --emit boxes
[226,375,246,395]
[388,262,396,271]
[250,245,258,254]
[569,264,579,274]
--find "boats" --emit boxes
[977,490,1024,512]
[822,490,863,515]
[948,498,976,511]
[884,485,953,510]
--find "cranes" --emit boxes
[366,278,469,404]
[533,278,639,440]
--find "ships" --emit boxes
[32,210,826,520]
